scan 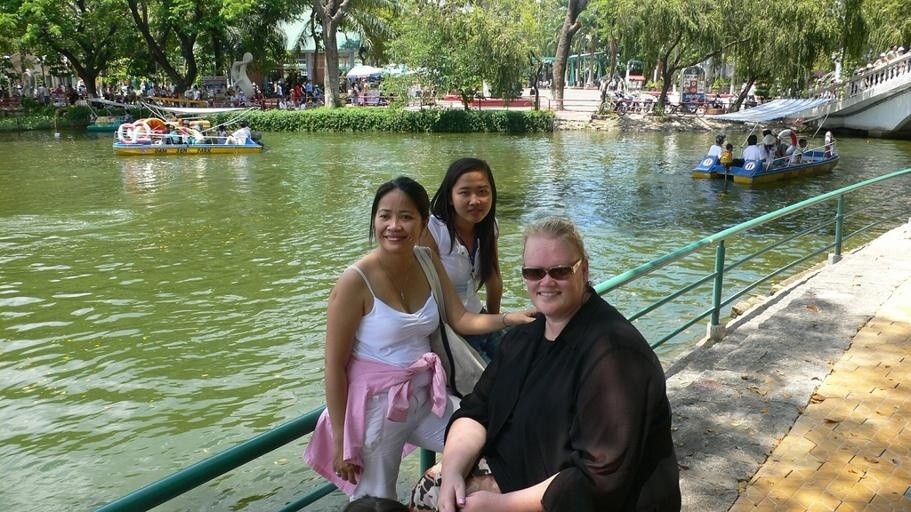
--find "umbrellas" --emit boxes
[346,62,441,81]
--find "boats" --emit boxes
[83,95,137,130]
[691,97,840,184]
[111,97,263,153]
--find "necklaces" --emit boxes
[386,265,415,303]
[452,224,483,314]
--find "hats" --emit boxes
[714,134,725,143]
[763,134,776,145]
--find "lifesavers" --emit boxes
[824,131,833,157]
[133,120,151,143]
[118,124,134,144]
[776,130,797,155]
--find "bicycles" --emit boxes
[603,90,704,118]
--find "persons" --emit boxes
[350,78,440,107]
[708,133,728,161]
[722,142,735,166]
[408,214,684,511]
[714,94,723,109]
[16,78,325,113]
[759,128,808,170]
[428,154,505,318]
[808,39,911,106]
[743,135,768,167]
[303,173,548,503]
[150,120,254,145]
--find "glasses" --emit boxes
[521,259,582,280]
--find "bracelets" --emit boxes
[503,311,511,327]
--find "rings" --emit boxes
[336,471,341,474]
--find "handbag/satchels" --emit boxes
[431,322,487,398]
